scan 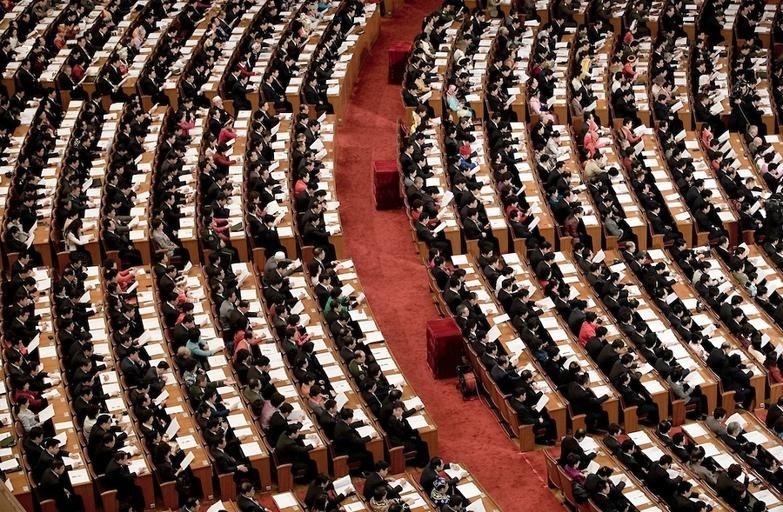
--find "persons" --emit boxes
[0,0,783,511]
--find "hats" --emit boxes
[275,250,286,260]
[211,95,222,103]
